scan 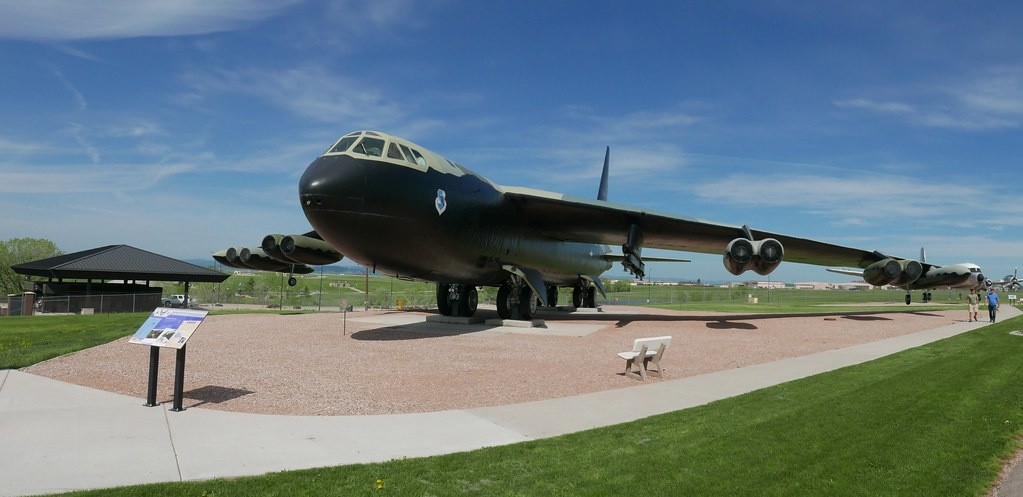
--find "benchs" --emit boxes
[617,335,673,384]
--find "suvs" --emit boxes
[163,295,191,307]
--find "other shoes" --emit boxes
[974,315,978,320]
[990,319,995,322]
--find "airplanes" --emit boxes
[826,246,1023,307]
[209,129,972,320]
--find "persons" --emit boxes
[984,287,1000,323]
[966,289,979,322]
